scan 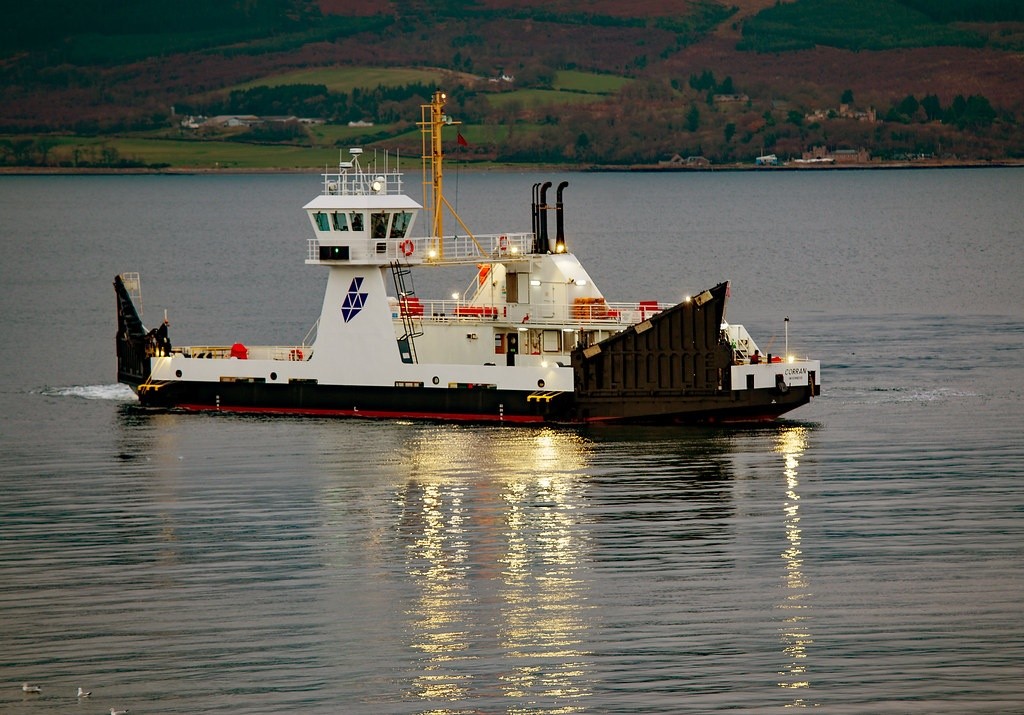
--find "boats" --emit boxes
[111,90,823,428]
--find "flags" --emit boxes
[458,133,468,147]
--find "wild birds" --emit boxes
[77,687,92,698]
[107,707,130,715]
[21,681,43,692]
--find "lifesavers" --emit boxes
[500,237,509,250]
[289,349,303,361]
[402,240,414,255]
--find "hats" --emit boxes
[164,320,169,323]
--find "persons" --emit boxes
[750,349,759,364]
[352,217,361,231]
